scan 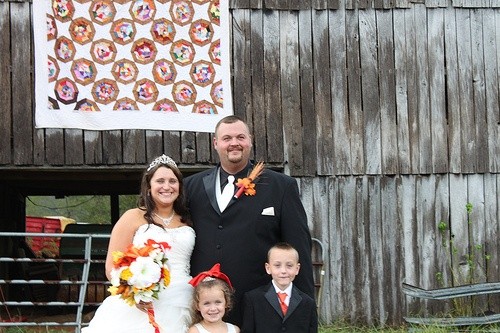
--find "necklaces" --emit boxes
[147,203,181,233]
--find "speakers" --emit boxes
[59,223,116,281]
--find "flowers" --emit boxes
[106,239,172,333]
[234,159,265,198]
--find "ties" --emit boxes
[222,176,235,210]
[278,292,288,316]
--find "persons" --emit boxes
[242,243,319,333]
[80,155,196,333]
[175,115,319,333]
[186,263,240,333]
